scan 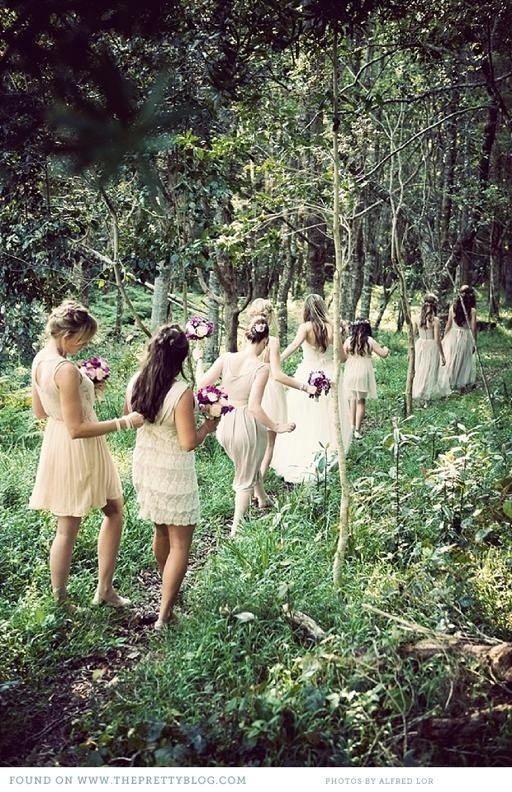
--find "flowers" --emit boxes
[182,315,217,342]
[193,384,236,420]
[305,369,331,400]
[75,354,114,386]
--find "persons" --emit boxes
[269,294,353,484]
[411,294,446,408]
[31,300,145,609]
[192,319,296,537]
[343,318,389,439]
[243,298,317,497]
[123,326,221,631]
[441,285,477,395]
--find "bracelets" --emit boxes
[203,423,211,433]
[300,384,307,391]
[114,416,135,432]
[195,357,202,367]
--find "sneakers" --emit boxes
[423,402,430,409]
[259,495,274,507]
[93,585,133,606]
[355,431,363,439]
